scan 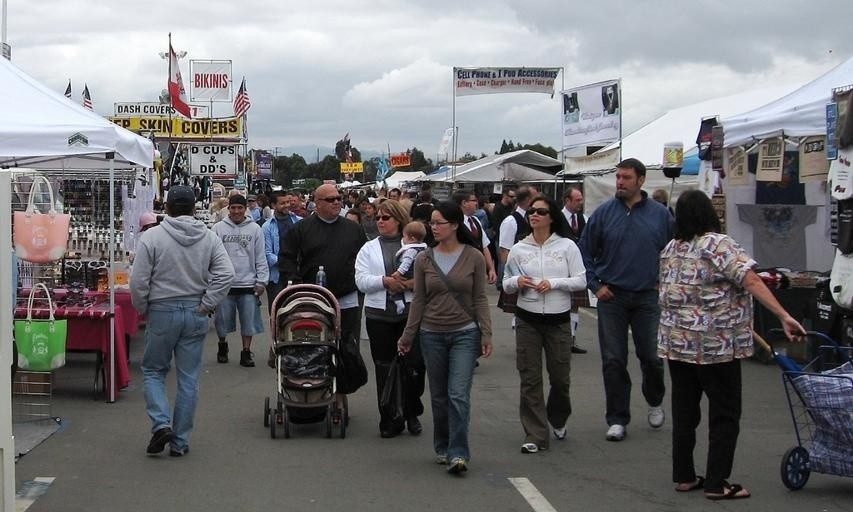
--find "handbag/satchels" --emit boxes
[15,320,67,371]
[13,211,71,263]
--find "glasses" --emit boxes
[65,260,82,272]
[88,260,106,270]
[319,195,549,226]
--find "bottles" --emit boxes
[286,280,292,286]
[316,266,327,288]
[98,268,108,292]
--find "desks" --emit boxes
[12,304,132,400]
[18,286,145,363]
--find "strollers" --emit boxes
[263,282,349,440]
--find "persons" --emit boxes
[577,157,677,441]
[658,189,806,500]
[138,209,164,233]
[128,186,235,459]
[652,188,675,216]
[397,202,493,474]
[353,200,425,438]
[212,184,587,366]
[501,195,589,452]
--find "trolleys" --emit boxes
[767,326,852,491]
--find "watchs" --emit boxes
[67,225,123,261]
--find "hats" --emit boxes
[138,186,257,231]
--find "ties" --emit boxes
[468,218,479,238]
[572,215,577,234]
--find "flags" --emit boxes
[63,84,71,98]
[83,85,94,113]
[232,82,251,120]
[169,45,192,120]
